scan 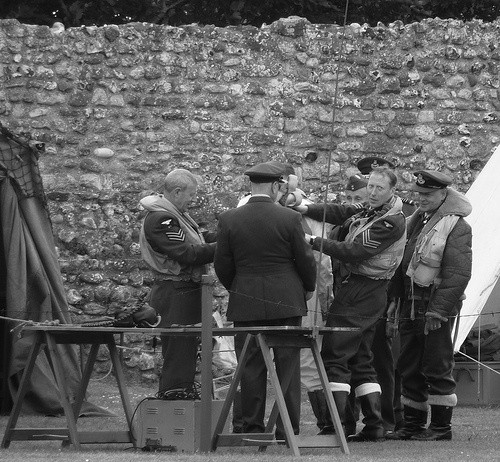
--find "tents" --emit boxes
[0,121,114,417]
[445,143,499,356]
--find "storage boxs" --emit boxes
[452,362,500,406]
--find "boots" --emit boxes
[347,383,385,442]
[308,390,326,430]
[411,394,458,441]
[384,395,429,440]
[315,381,351,436]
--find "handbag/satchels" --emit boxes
[113,306,161,329]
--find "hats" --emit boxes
[357,156,395,178]
[410,170,452,194]
[345,174,367,192]
[244,160,285,184]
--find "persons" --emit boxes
[141,169,217,399]
[215,158,473,442]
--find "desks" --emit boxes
[1,323,361,456]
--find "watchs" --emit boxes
[309,235,317,245]
